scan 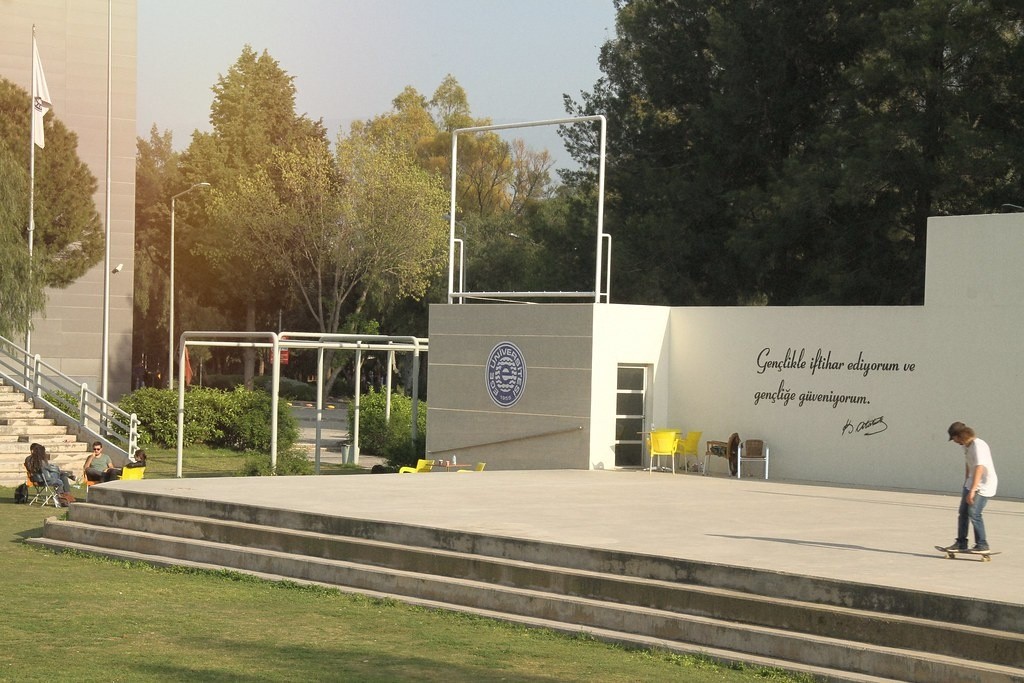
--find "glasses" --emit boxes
[92,448,102,450]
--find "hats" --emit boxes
[948,422,965,442]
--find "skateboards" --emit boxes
[934,545,1003,562]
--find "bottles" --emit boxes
[452,453,456,466]
[650,423,655,432]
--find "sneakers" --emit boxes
[968,543,990,553]
[946,539,968,551]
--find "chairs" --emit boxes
[737,439,770,480]
[645,430,679,474]
[674,430,703,473]
[701,433,741,476]
[23,463,51,506]
[116,465,146,481]
[456,462,487,473]
[399,458,435,474]
[28,464,65,508]
[83,466,101,498]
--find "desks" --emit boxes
[636,431,684,471]
[425,463,472,472]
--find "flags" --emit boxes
[31,33,50,152]
[185,347,193,389]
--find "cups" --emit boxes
[446,461,450,466]
[438,460,443,466]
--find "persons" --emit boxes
[25,443,72,499]
[84,442,115,483]
[947,422,998,554]
[106,450,147,480]
[30,446,76,503]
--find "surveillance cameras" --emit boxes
[114,263,123,274]
